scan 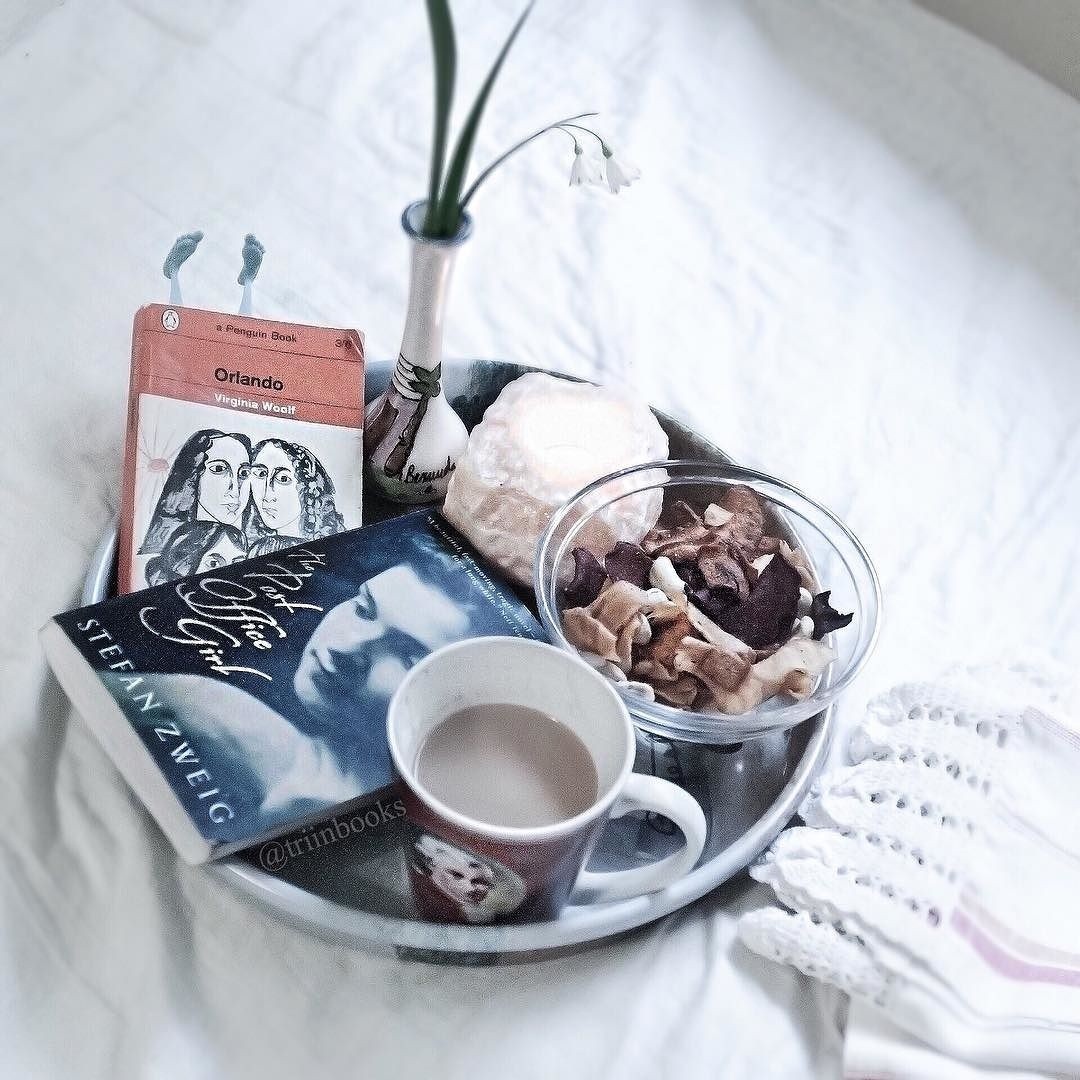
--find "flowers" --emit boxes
[365,0,638,240]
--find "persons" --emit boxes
[82,533,514,856]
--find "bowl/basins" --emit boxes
[532,458,884,746]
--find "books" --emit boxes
[118,302,367,622]
[39,508,543,862]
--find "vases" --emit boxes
[366,202,473,506]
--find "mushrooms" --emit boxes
[562,488,853,718]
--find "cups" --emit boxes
[385,634,709,927]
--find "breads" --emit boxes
[442,371,669,591]
